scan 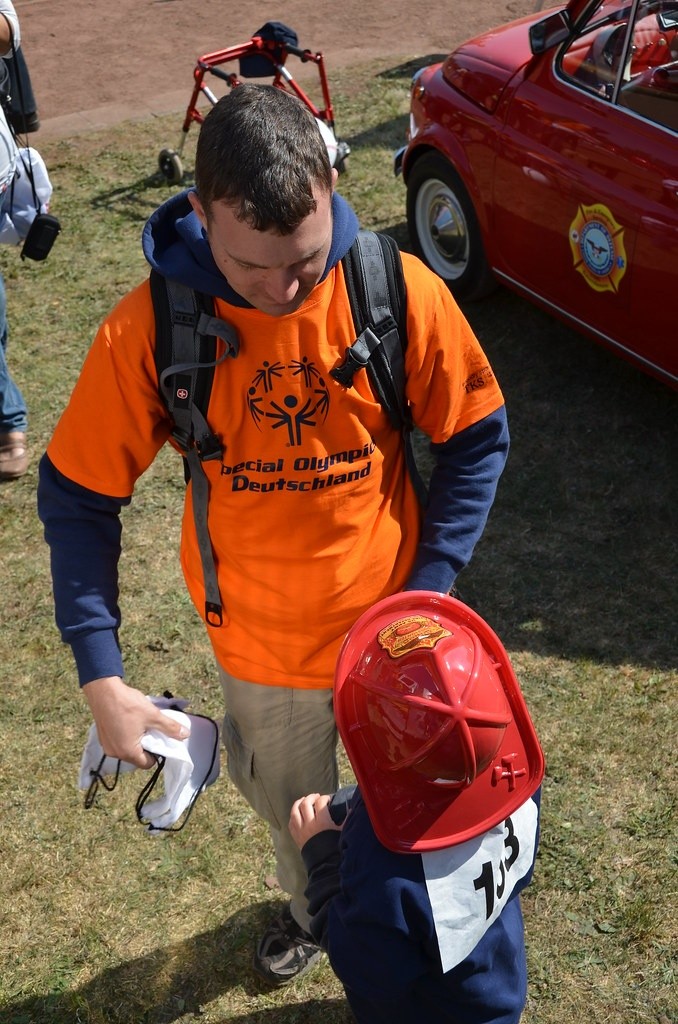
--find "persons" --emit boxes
[287,590,544,1024]
[37,90,510,986]
[0,0,28,480]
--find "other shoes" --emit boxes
[0,432,28,478]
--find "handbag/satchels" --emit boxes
[0,147,61,261]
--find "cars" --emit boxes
[393,0,678,387]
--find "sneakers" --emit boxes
[252,906,324,985]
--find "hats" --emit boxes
[239,23,298,78]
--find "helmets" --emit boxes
[334,591,545,852]
